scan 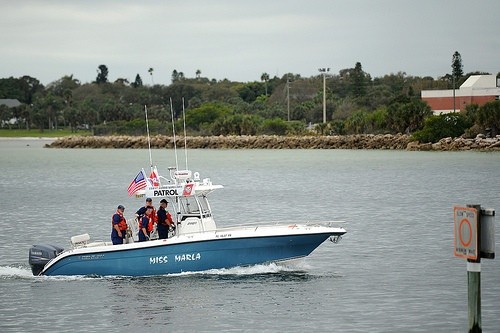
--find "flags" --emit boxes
[182,184,194,197]
[127,171,147,198]
[149,172,159,187]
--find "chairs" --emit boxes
[128,218,139,242]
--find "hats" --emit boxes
[118,204,126,208]
[160,199,168,203]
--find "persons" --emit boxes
[111,205,127,245]
[135,198,155,225]
[138,206,154,242]
[156,199,173,239]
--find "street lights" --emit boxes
[318,68,330,124]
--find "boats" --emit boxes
[28,98,347,277]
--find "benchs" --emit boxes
[70,232,106,248]
